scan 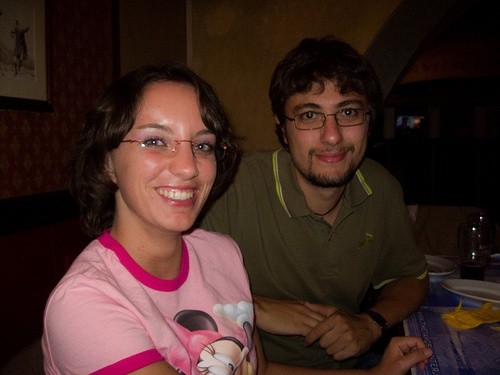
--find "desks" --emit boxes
[401,257,500,375]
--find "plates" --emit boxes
[439,279,500,311]
[424,254,458,282]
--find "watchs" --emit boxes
[359,310,388,336]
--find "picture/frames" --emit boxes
[0,0,54,113]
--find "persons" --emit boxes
[40,60,433,375]
[192,36,430,369]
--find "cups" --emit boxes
[457,212,495,281]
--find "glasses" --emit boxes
[121,135,220,157]
[283,108,371,130]
